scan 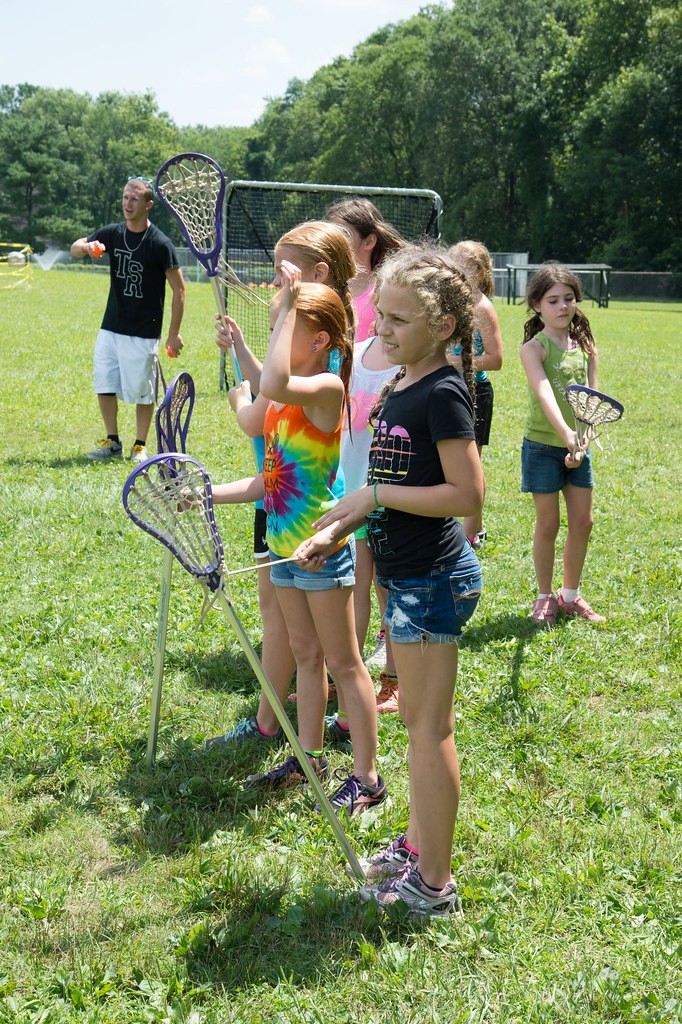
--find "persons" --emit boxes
[172,195,502,816]
[70,178,185,463]
[520,264,606,626]
[290,247,486,920]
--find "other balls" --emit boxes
[91,245,103,257]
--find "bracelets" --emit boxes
[81,242,86,254]
[372,481,382,509]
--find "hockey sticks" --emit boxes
[144,371,200,773]
[121,449,370,887]
[155,151,246,386]
[564,384,624,460]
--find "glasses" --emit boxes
[127,175,156,193]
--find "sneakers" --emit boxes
[531,595,607,627]
[464,533,487,552]
[325,714,352,752]
[130,445,149,463]
[201,717,285,753]
[346,836,465,923]
[88,438,125,462]
[363,628,387,667]
[287,680,335,702]
[376,671,399,713]
[313,769,392,834]
[241,752,328,796]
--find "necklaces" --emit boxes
[123,224,148,251]
